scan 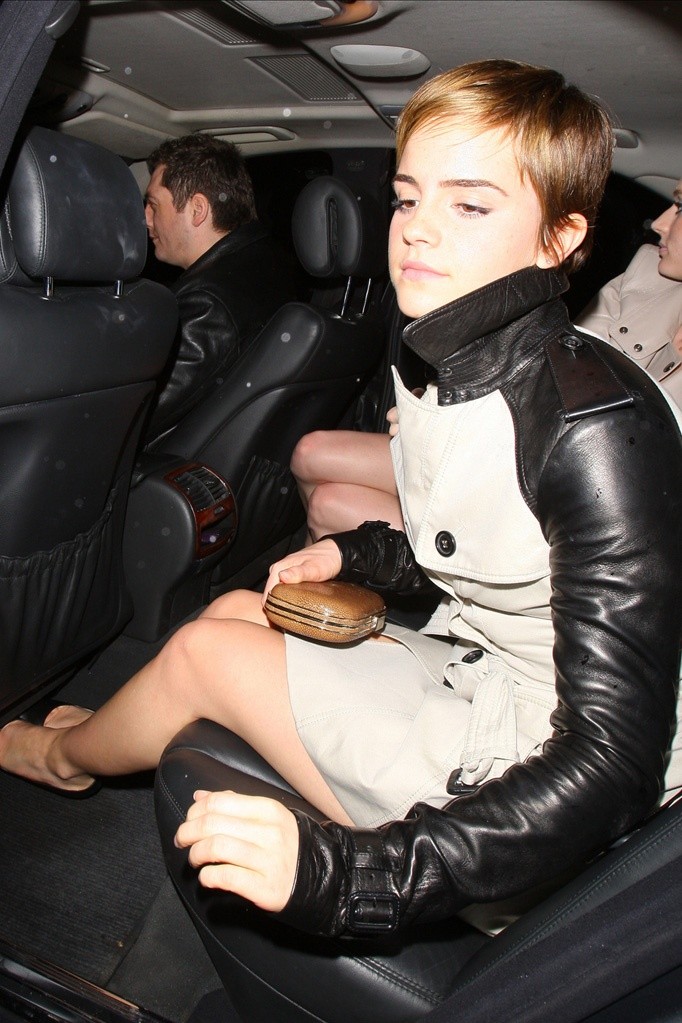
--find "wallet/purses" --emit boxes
[265,577,388,643]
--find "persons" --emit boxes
[133,131,289,452]
[585,178,682,416]
[0,56,680,955]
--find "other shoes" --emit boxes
[7,778,101,800]
[19,698,95,727]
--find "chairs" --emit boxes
[177,179,373,592]
[0,129,181,740]
[153,711,682,1022]
